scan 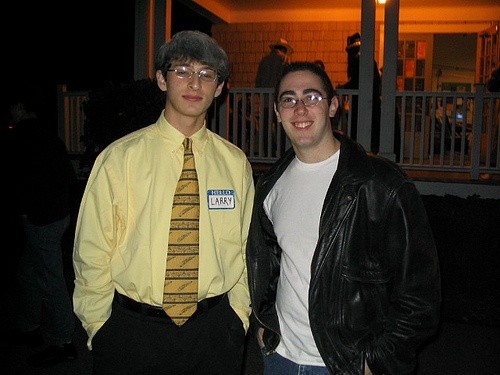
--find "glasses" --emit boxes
[276,94,328,109]
[167,65,222,82]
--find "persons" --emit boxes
[314,61,326,71]
[254,38,295,156]
[480,66,500,186]
[244,60,441,375]
[336,33,401,152]
[70,30,256,375]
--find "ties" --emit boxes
[162,138,200,326]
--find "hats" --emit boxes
[345,32,361,52]
[268,38,292,52]
